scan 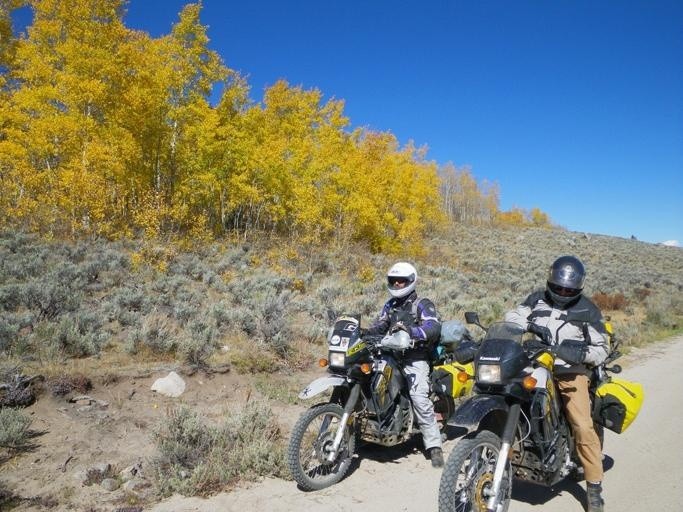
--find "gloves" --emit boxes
[389,321,411,336]
[528,321,552,340]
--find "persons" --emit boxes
[504,256,613,512]
[360,263,444,467]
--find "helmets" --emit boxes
[546,256,586,305]
[385,262,418,299]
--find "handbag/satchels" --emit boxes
[438,320,473,346]
[432,360,478,400]
[592,378,644,434]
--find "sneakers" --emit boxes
[586,481,603,512]
[430,448,444,466]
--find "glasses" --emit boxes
[551,283,576,293]
[389,277,406,284]
